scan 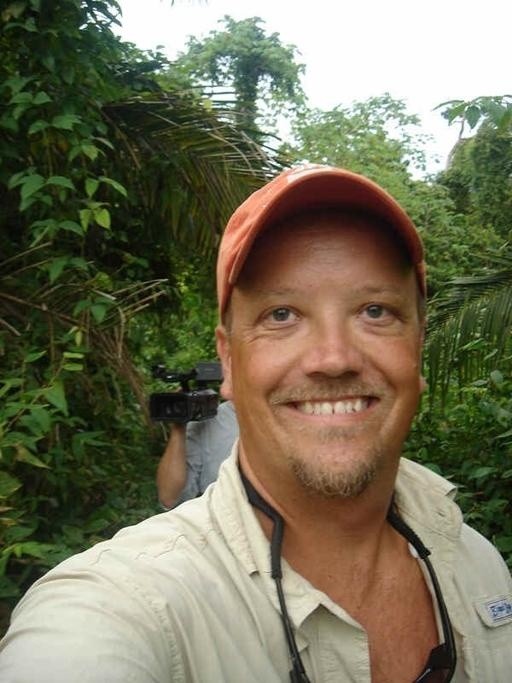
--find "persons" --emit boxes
[0,165,512,683]
[156,387,239,511]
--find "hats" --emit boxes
[217,163,427,326]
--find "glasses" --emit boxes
[275,558,458,683]
